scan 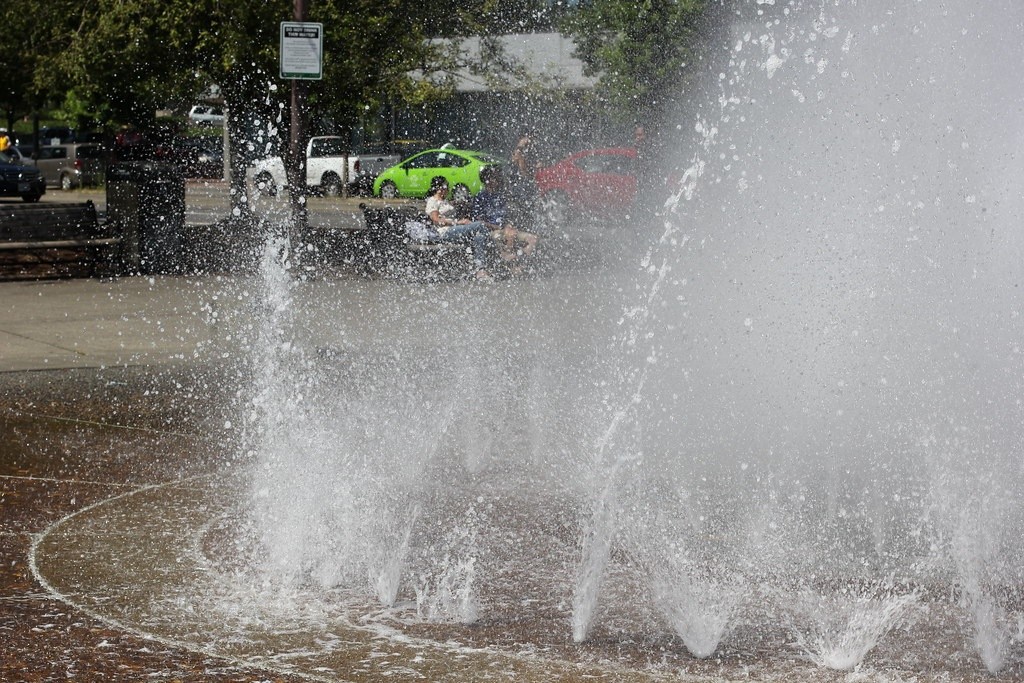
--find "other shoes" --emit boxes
[468,273,493,286]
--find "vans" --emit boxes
[30,142,105,190]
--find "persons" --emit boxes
[633,124,683,219]
[427,128,546,284]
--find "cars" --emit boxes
[371,143,515,203]
[188,105,224,127]
[1,146,49,202]
[535,146,672,226]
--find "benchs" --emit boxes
[0,199,125,280]
[358,202,468,281]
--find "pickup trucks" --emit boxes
[248,134,401,199]
